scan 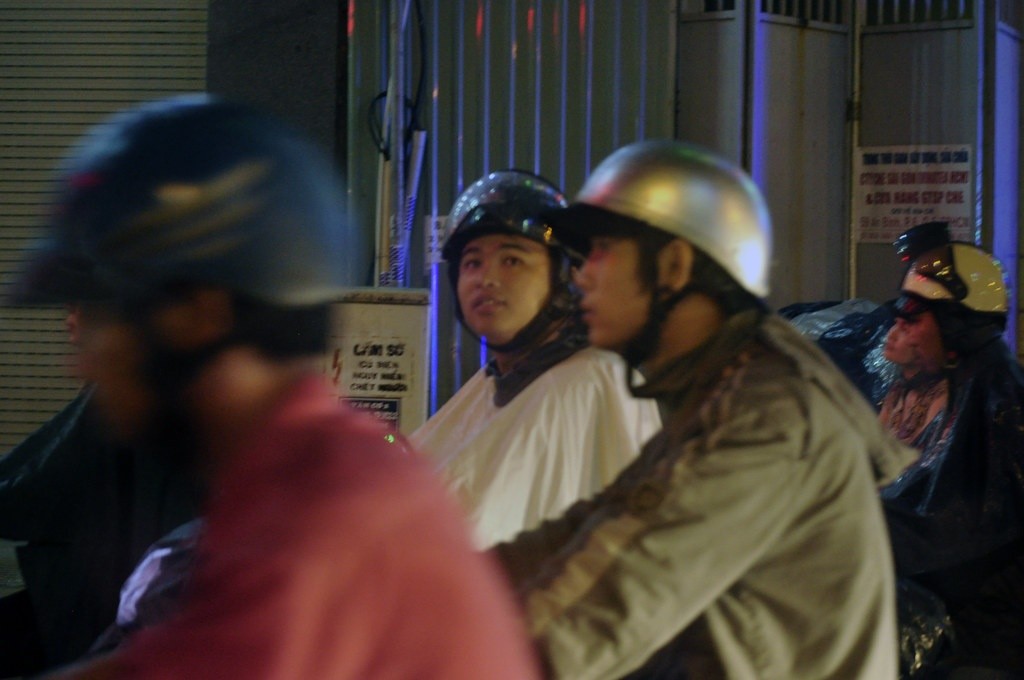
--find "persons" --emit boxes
[1,90,902,680]
[877,243,1024,680]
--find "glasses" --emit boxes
[912,245,967,298]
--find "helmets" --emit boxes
[901,242,1009,331]
[48,93,354,309]
[537,138,771,314]
[440,167,572,289]
